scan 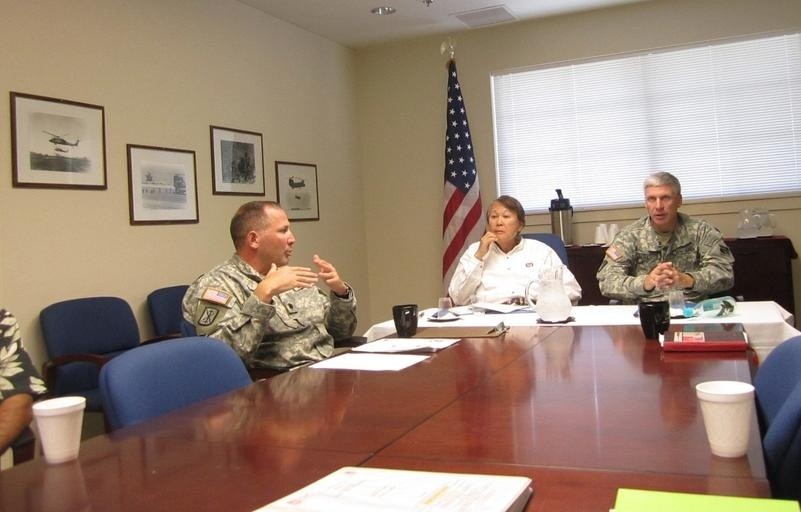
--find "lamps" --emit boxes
[371,8,396,17]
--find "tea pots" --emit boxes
[525,277,573,323]
[549,189,574,248]
[759,206,777,237]
[736,206,762,237]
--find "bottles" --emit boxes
[682,296,736,319]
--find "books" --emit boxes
[663,322,748,352]
[251,466,533,512]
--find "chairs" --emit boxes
[39,297,151,417]
[515,232,568,269]
[747,336,800,491]
[147,285,193,345]
[98,337,252,436]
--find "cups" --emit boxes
[393,305,418,337]
[33,394,86,465]
[600,224,608,241]
[609,224,619,240]
[438,298,453,319]
[471,295,486,317]
[670,290,687,317]
[696,380,755,459]
[595,226,607,245]
[639,301,670,340]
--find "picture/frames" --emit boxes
[9,92,110,191]
[209,124,266,197]
[274,161,321,222]
[546,225,791,314]
[125,143,201,225]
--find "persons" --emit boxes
[448,195,582,307]
[0,304,49,457]
[597,172,735,305]
[180,201,358,383]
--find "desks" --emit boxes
[2,303,770,512]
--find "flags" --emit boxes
[442,37,486,297]
